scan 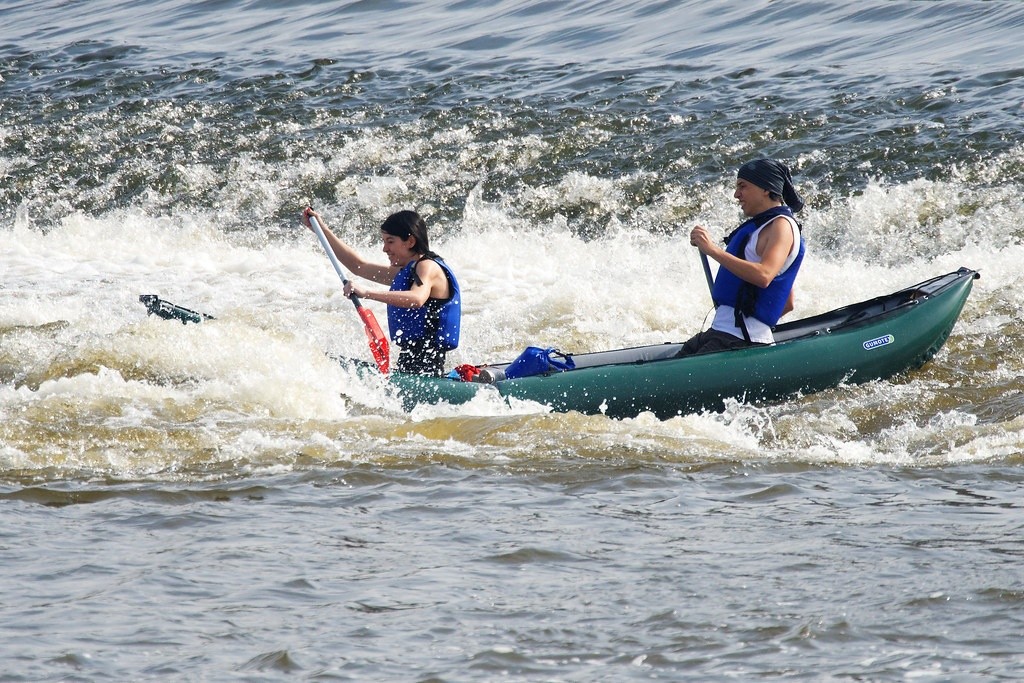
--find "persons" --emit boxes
[302,206,464,378]
[674,157,805,358]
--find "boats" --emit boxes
[137,267,981,424]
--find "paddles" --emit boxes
[695,243,716,303]
[306,206,391,375]
